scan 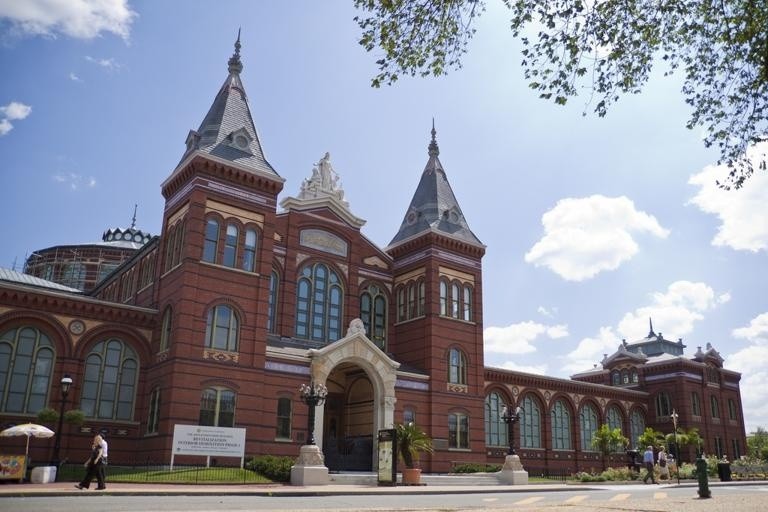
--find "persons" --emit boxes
[654,445,674,485]
[95,432,109,488]
[73,435,106,490]
[641,444,659,484]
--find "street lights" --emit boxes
[298,381,328,445]
[500,403,522,454]
[669,409,679,484]
[51,375,73,465]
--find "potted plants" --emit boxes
[390,422,432,485]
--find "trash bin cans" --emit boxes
[717,463,732,481]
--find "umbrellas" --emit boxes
[0,419,54,458]
